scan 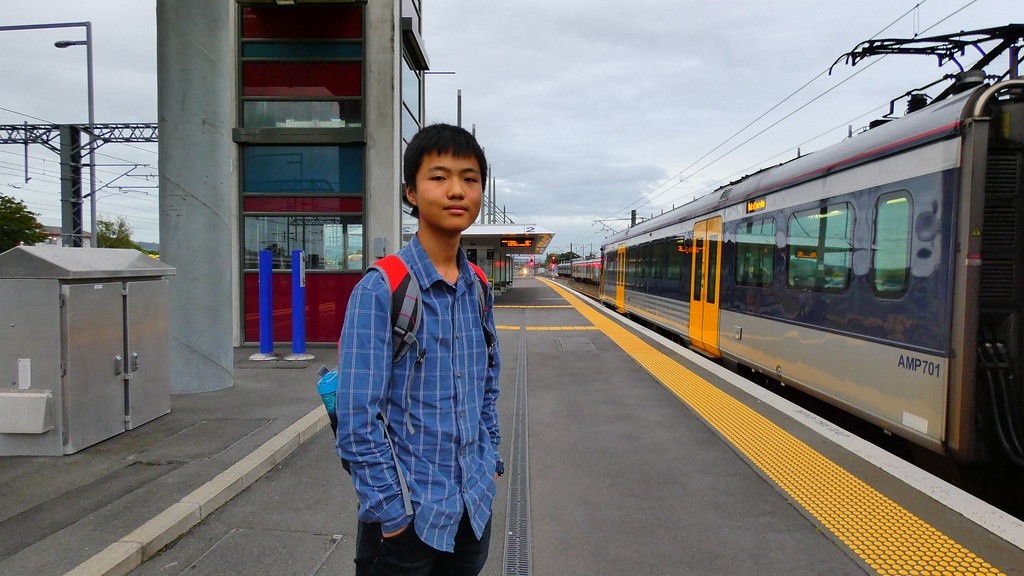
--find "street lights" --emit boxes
[53,38,98,250]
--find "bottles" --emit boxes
[317,370,339,423]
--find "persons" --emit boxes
[336,123,504,576]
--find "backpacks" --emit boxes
[318,254,491,476]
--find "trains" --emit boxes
[557,259,602,286]
[599,80,1024,507]
